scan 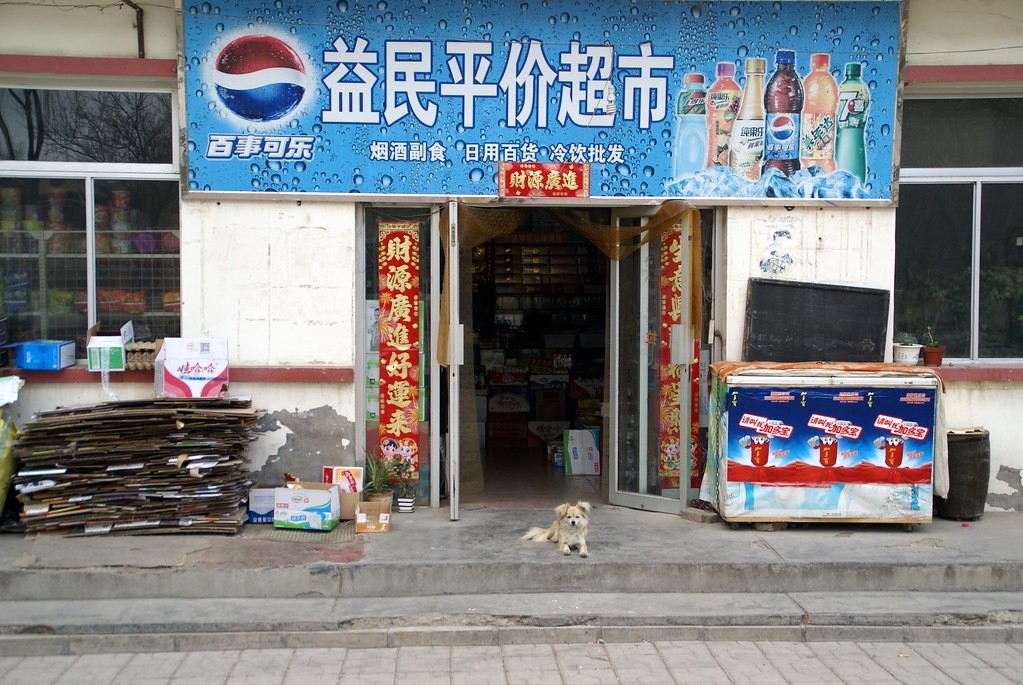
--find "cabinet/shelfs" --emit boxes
[0,230,181,369]
[484,243,605,367]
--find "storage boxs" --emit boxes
[86,320,134,372]
[473,331,658,486]
[365,300,429,506]
[0,339,75,371]
[248,467,390,532]
[154,338,229,398]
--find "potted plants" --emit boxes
[922,325,945,367]
[361,446,393,511]
[392,457,416,512]
[893,332,923,366]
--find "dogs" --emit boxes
[520,500,591,557]
